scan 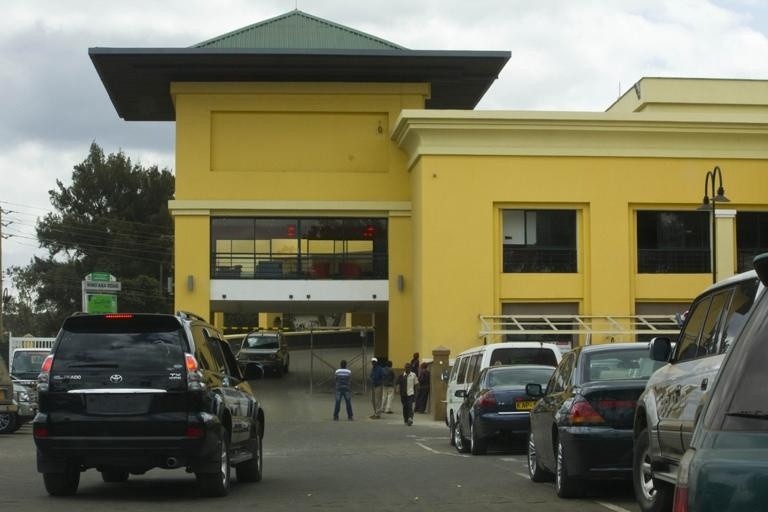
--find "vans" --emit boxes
[0,347,53,435]
[445,341,563,444]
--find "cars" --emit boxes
[528,342,675,497]
[453,365,556,456]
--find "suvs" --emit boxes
[631,251,767,511]
[31,311,265,497]
[236,330,290,379]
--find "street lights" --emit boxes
[695,166,731,283]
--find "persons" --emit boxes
[414,361,431,414]
[394,362,421,426]
[368,357,385,419]
[409,352,419,377]
[331,359,354,422]
[380,360,400,415]
